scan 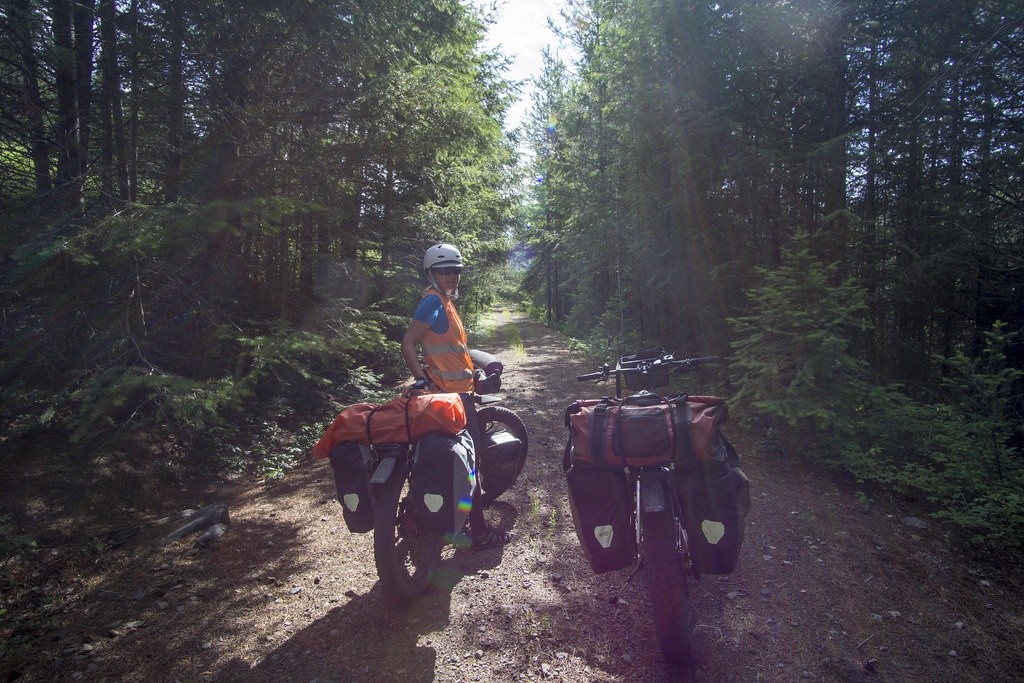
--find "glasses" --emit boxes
[431,267,461,276]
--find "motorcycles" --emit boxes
[577,346,718,658]
[369,348,529,606]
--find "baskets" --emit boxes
[620,346,670,392]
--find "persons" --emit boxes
[401,244,512,549]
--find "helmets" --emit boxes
[423,244,464,278]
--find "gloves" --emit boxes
[405,376,430,390]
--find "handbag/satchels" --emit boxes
[566,468,634,575]
[681,467,751,575]
[406,429,477,537]
[328,440,375,533]
[563,390,740,474]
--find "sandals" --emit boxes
[472,532,514,552]
